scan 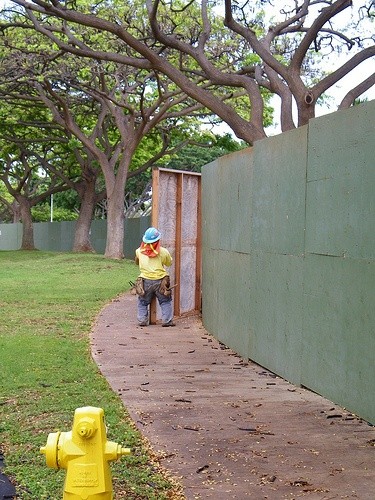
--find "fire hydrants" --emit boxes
[38,406,132,500]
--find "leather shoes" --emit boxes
[162,323,176,327]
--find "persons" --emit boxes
[128,227,179,327]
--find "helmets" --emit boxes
[142,227,161,243]
